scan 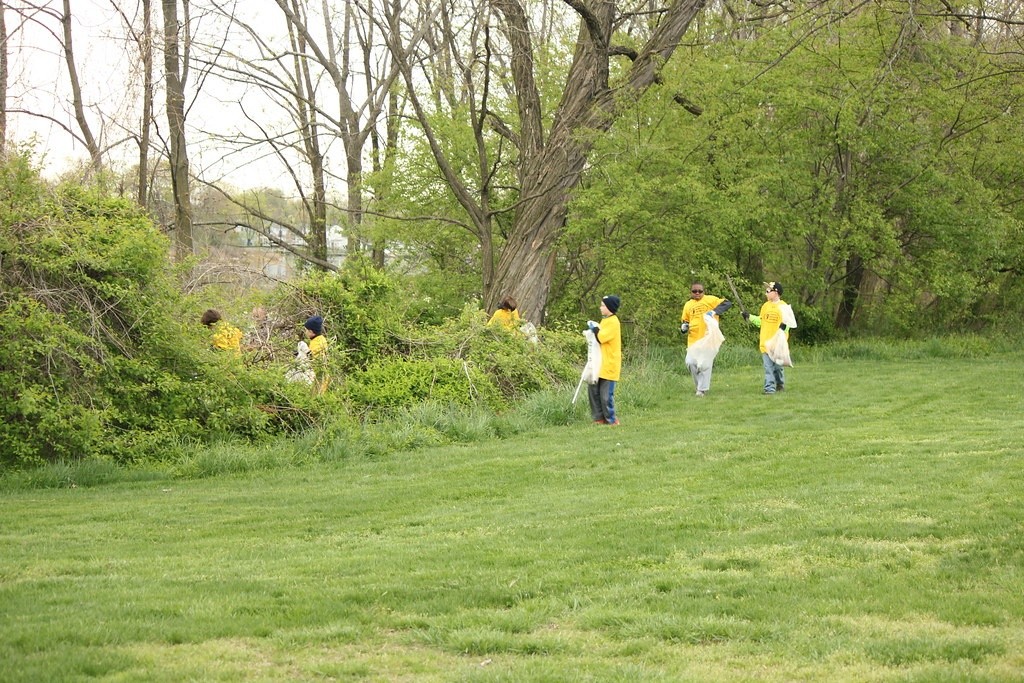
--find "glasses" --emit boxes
[766,290,774,293]
[692,290,703,294]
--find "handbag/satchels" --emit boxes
[765,328,794,368]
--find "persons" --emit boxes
[586,295,622,427]
[681,283,733,398]
[484,295,529,336]
[200,308,243,359]
[741,281,790,394]
[294,315,329,394]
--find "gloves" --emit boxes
[589,322,594,330]
[779,323,786,331]
[706,311,716,318]
[742,311,749,319]
[681,322,689,332]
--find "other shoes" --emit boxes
[695,390,705,397]
[593,418,606,424]
[610,420,620,426]
[776,384,784,392]
[764,391,775,394]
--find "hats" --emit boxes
[763,282,783,295]
[305,316,322,331]
[602,296,620,314]
